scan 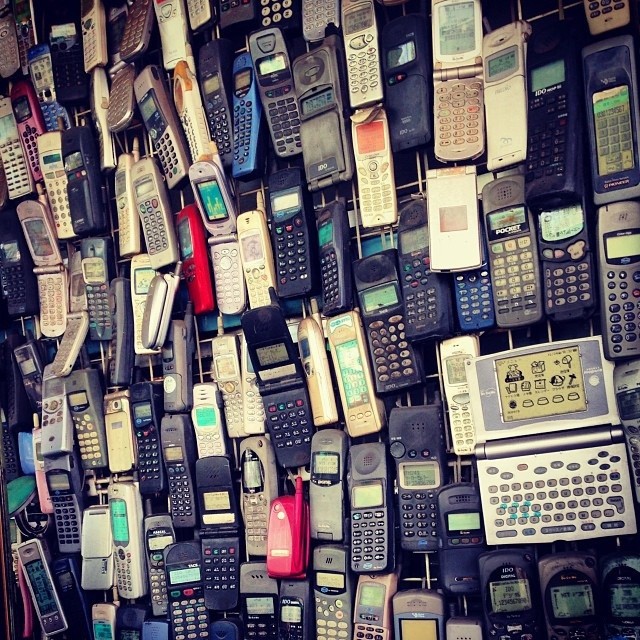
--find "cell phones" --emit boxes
[266,477,311,580]
[389,390,449,553]
[188,160,248,316]
[41,363,74,457]
[190,383,232,459]
[103,388,138,474]
[80,0,108,73]
[153,0,194,71]
[0,97,36,200]
[37,131,80,239]
[80,236,117,341]
[0,406,21,482]
[11,80,47,182]
[428,436,430,438]
[381,0,433,153]
[482,0,533,172]
[46,19,89,111]
[90,66,116,175]
[173,58,218,164]
[278,580,316,640]
[117,604,152,640]
[239,420,284,556]
[106,0,142,132]
[482,174,544,328]
[134,64,194,190]
[537,552,602,640]
[195,456,244,611]
[16,539,68,637]
[258,0,301,33]
[44,452,88,553]
[241,287,316,468]
[446,603,483,640]
[160,413,200,528]
[397,199,454,344]
[51,251,90,378]
[454,199,495,332]
[13,329,48,417]
[297,297,339,427]
[600,552,640,640]
[432,1,486,164]
[0,204,39,316]
[353,559,403,640]
[290,23,356,191]
[595,201,640,361]
[162,519,211,640]
[186,0,220,35]
[352,248,427,398]
[80,505,115,592]
[240,328,268,435]
[143,498,177,616]
[31,413,55,514]
[240,561,280,640]
[317,196,353,316]
[436,482,488,594]
[439,335,481,456]
[236,191,278,310]
[538,186,597,321]
[582,35,640,205]
[477,545,546,640]
[114,137,142,257]
[110,265,135,386]
[249,25,302,159]
[174,190,218,316]
[142,603,171,640]
[211,316,251,438]
[326,311,387,438]
[582,0,632,36]
[120,0,155,64]
[91,587,121,640]
[129,253,161,355]
[15,0,39,77]
[0,0,21,79]
[232,53,269,183]
[341,0,384,109]
[309,424,351,541]
[392,589,446,640]
[612,360,640,504]
[523,21,587,211]
[242,333,266,434]
[347,443,396,574]
[302,0,340,43]
[312,518,358,640]
[425,165,486,275]
[0,333,31,431]
[16,200,69,338]
[26,43,73,132]
[210,617,244,640]
[130,157,180,271]
[50,555,93,640]
[107,472,150,598]
[129,381,169,494]
[9,516,23,589]
[351,108,398,229]
[162,300,195,414]
[141,260,183,351]
[197,31,234,167]
[65,369,108,471]
[61,126,104,235]
[269,157,321,300]
[220,0,258,32]
